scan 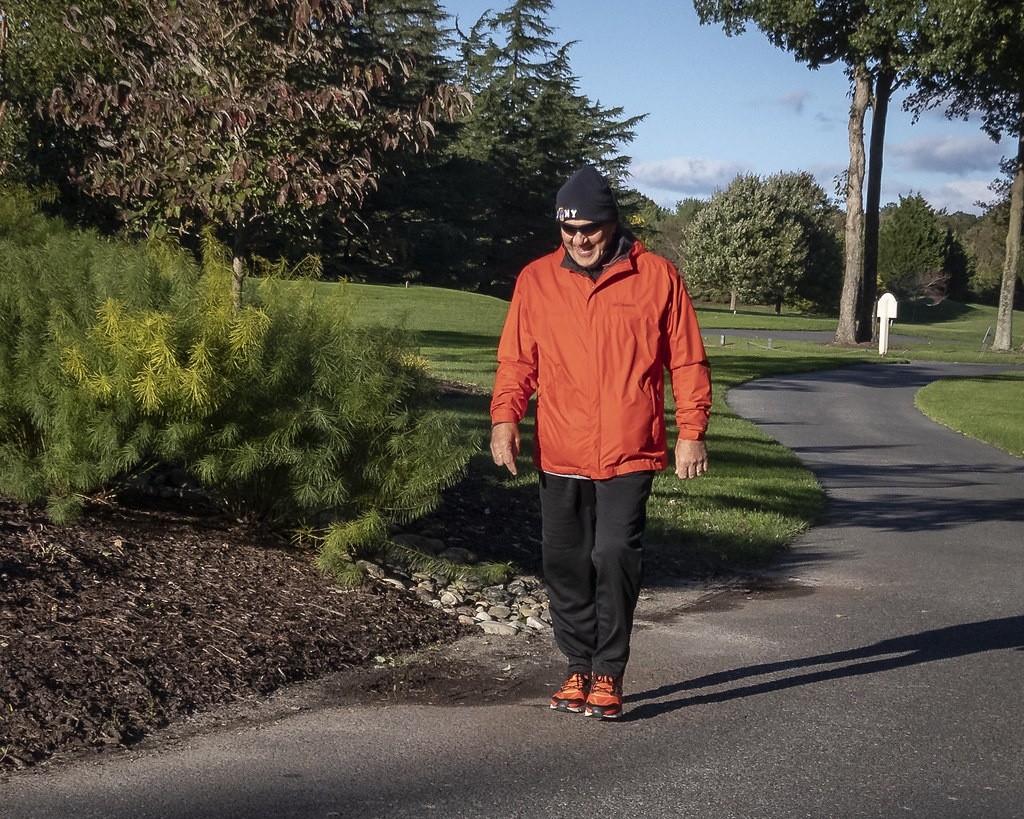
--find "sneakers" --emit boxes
[550,671,623,718]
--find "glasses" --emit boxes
[561,222,607,236]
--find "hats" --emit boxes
[555,166,619,221]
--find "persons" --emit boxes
[488,166,714,721]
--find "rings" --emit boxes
[696,469,704,474]
